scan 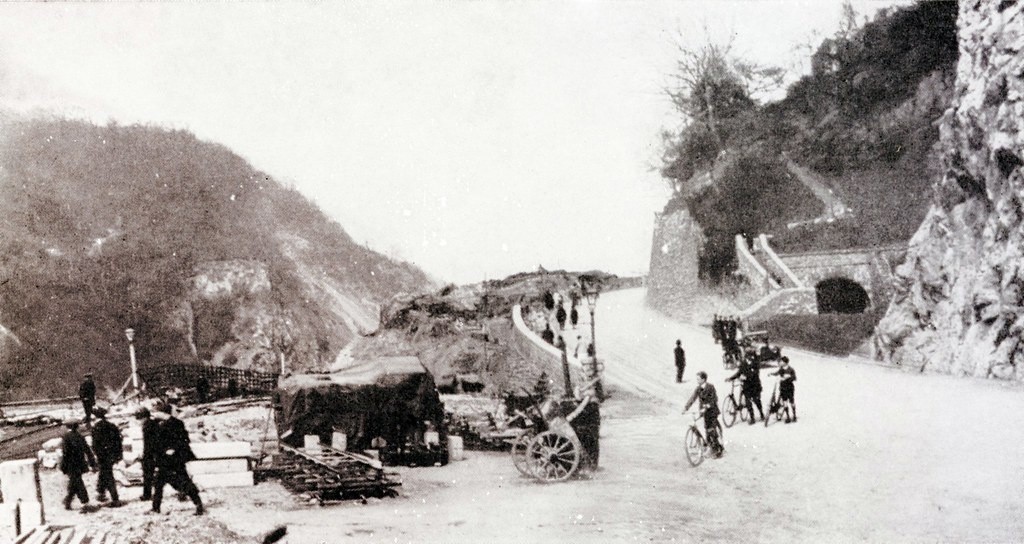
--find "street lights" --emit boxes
[586,289,602,402]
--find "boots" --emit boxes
[784,406,791,423]
[792,406,796,421]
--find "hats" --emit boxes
[91,408,107,421]
[65,420,79,429]
[134,408,151,419]
[154,403,172,414]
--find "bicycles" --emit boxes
[681,410,724,467]
[764,373,785,427]
[722,379,748,428]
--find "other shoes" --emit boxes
[176,494,188,502]
[193,510,203,515]
[712,443,724,457]
[749,419,755,425]
[105,501,122,508]
[140,495,151,500]
[80,504,95,513]
[95,494,108,502]
[759,416,765,421]
[62,500,71,510]
[144,508,160,514]
[83,417,91,423]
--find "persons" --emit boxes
[61,372,248,516]
[676,314,796,458]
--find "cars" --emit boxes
[737,330,780,369]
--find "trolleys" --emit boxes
[511,387,599,484]
